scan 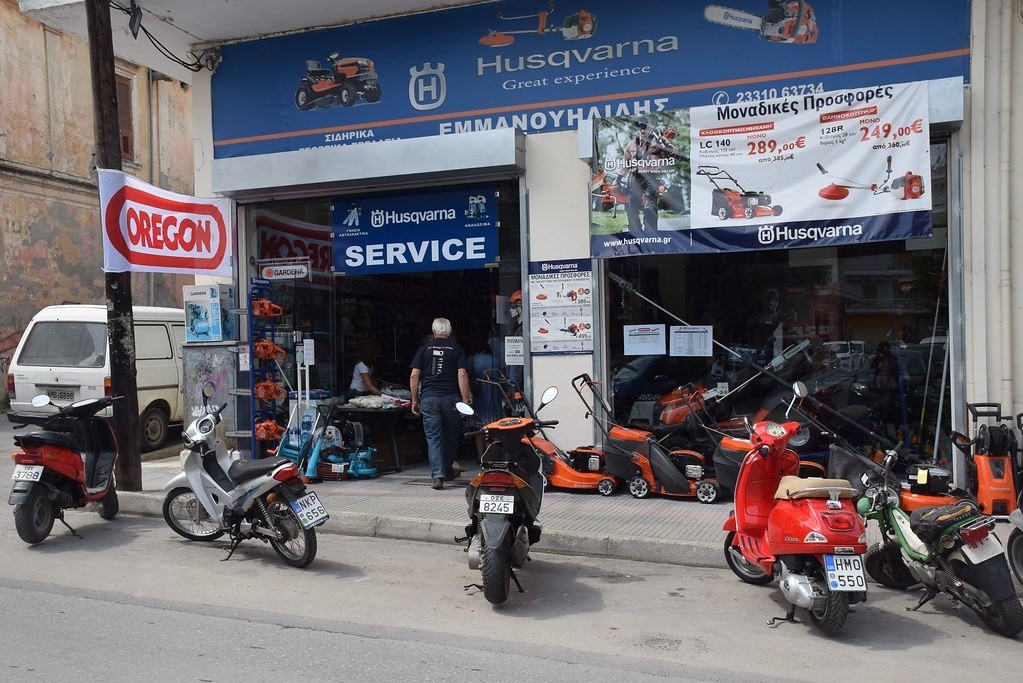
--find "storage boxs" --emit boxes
[371,432,424,466]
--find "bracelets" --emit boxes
[412,402,417,404]
[378,392,385,396]
[467,395,473,398]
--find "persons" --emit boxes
[871,338,903,435]
[466,334,502,466]
[350,337,397,397]
[409,317,473,488]
[790,332,837,402]
[487,331,507,356]
[615,114,678,232]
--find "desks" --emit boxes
[336,407,427,473]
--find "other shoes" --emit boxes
[447,469,460,481]
[431,478,443,490]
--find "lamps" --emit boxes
[205,48,223,72]
[129,0,143,40]
